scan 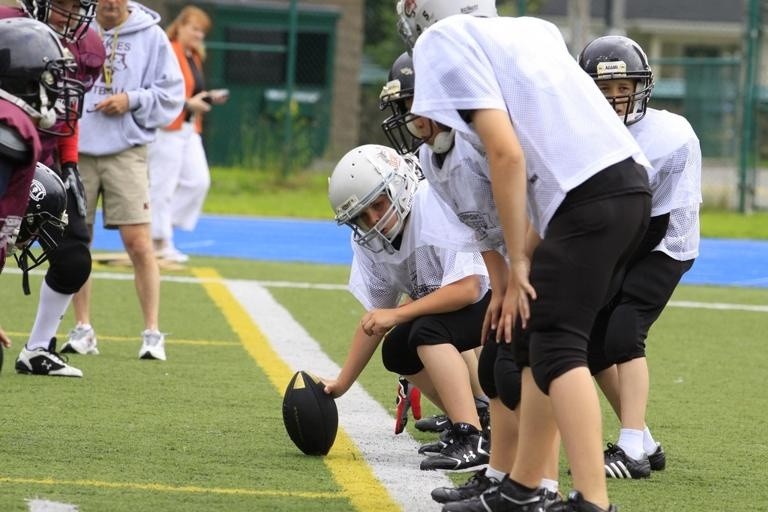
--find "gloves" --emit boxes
[395,374,421,434]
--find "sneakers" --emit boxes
[59,326,99,354]
[15,338,83,378]
[137,330,167,360]
[415,405,666,512]
[157,247,188,263]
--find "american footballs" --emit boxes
[283,370,338,456]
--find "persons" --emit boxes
[60,0,187,361]
[0,0,105,348]
[380,51,562,512]
[0,17,85,374]
[317,143,492,473]
[396,0,657,512]
[579,35,702,479]
[146,4,228,265]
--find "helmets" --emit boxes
[327,143,419,239]
[379,51,455,154]
[577,35,652,123]
[396,0,500,42]
[2,0,99,272]
